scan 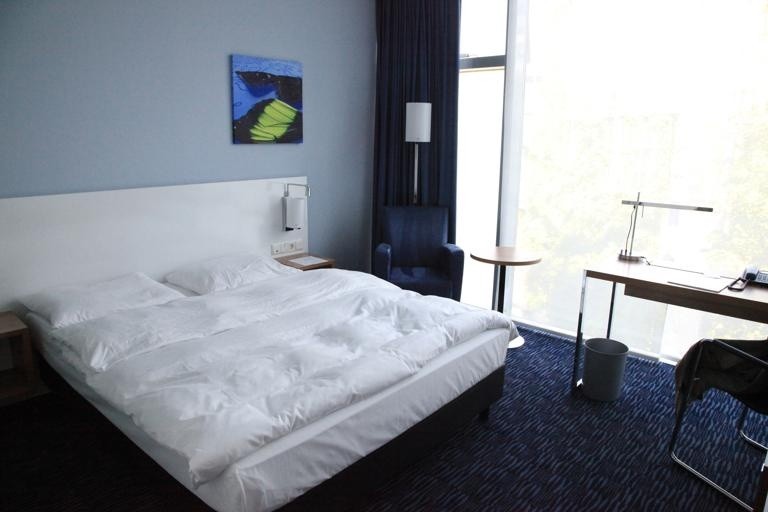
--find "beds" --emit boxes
[0,178,517,512]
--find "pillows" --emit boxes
[14,272,184,330]
[166,249,302,295]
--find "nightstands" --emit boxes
[0,311,34,404]
[273,253,336,270]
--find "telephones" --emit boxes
[742,267,768,287]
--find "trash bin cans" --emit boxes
[581,339,628,402]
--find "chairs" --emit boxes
[667,336,768,512]
[374,203,464,302]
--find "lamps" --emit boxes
[283,182,311,231]
[618,192,713,262]
[404,102,432,203]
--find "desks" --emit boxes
[570,261,768,392]
[470,247,544,348]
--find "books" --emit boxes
[668,261,740,292]
